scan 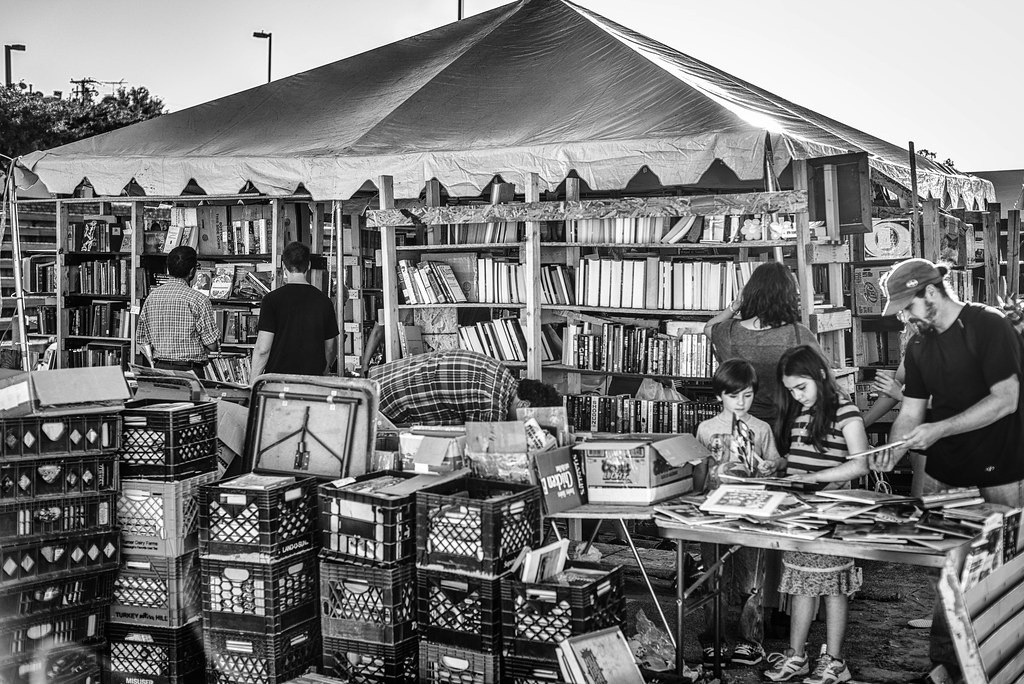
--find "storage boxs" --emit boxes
[0,397,718,684]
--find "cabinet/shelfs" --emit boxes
[57,190,978,577]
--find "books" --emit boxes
[21,205,1021,552]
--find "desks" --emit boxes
[653,522,948,684]
[544,494,700,648]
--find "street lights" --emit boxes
[253,32,271,84]
[4,43,26,88]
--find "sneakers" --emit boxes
[803,653,852,684]
[702,644,731,667]
[764,647,809,682]
[731,641,766,665]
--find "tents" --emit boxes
[8,0,1005,414]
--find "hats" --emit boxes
[883,260,943,317]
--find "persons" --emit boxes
[694,357,781,666]
[250,240,340,384]
[703,261,833,639]
[865,258,1024,683]
[131,246,220,379]
[763,345,869,683]
[363,348,562,428]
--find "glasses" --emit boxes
[189,262,201,270]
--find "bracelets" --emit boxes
[730,300,739,315]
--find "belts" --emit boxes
[159,360,202,368]
